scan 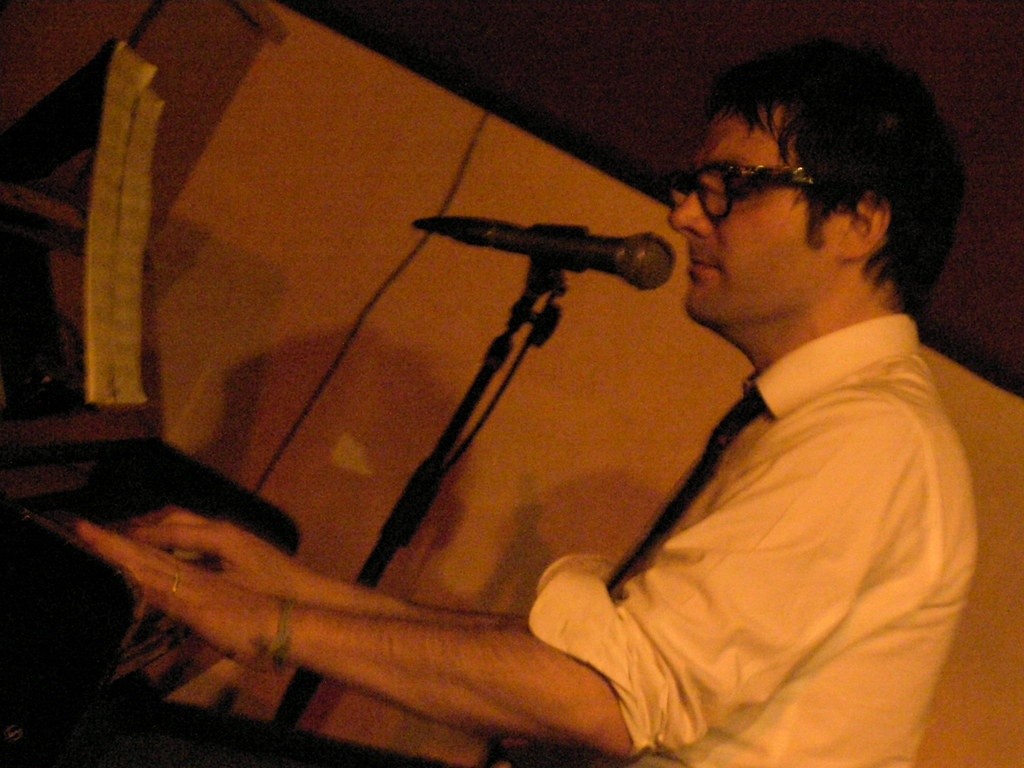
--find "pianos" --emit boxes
[0,0,305,768]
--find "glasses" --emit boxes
[668,162,861,221]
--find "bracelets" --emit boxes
[269,597,295,660]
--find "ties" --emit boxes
[482,386,770,767]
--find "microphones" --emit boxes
[411,215,676,292]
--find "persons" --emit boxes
[75,35,982,768]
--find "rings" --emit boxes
[172,573,179,592]
